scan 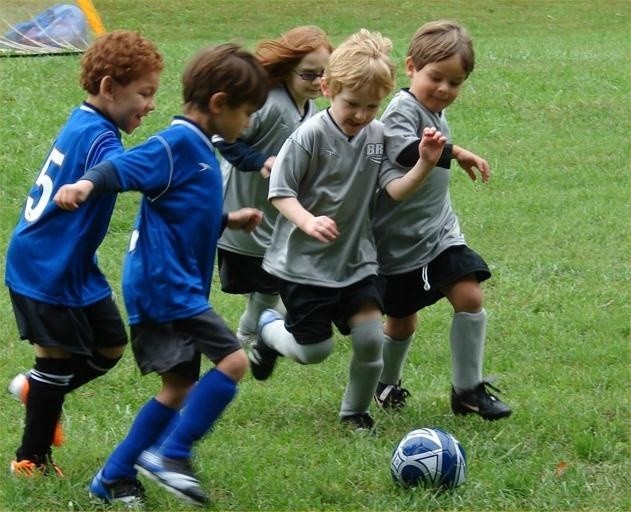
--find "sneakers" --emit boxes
[451,379,511,418]
[89,468,149,511]
[374,379,413,416]
[135,448,209,507]
[339,411,378,435]
[9,373,63,481]
[249,309,283,381]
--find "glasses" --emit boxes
[289,68,323,81]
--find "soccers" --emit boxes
[391,427,466,493]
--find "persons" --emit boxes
[247,29,448,434]
[212,25,334,348]
[6,31,163,480]
[52,44,266,511]
[374,20,514,421]
